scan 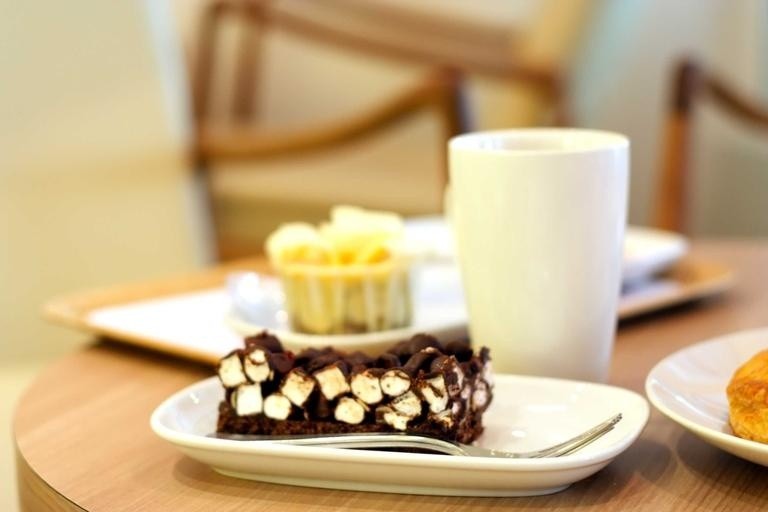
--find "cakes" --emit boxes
[265,205,416,333]
[216,335,493,454]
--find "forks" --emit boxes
[208,413,626,455]
[229,272,286,333]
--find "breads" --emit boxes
[728,350,768,445]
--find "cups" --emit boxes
[446,127,631,383]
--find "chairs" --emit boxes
[658,52,768,228]
[160,1,575,267]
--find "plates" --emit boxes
[229,324,470,353]
[408,220,688,288]
[645,326,768,464]
[149,371,650,496]
